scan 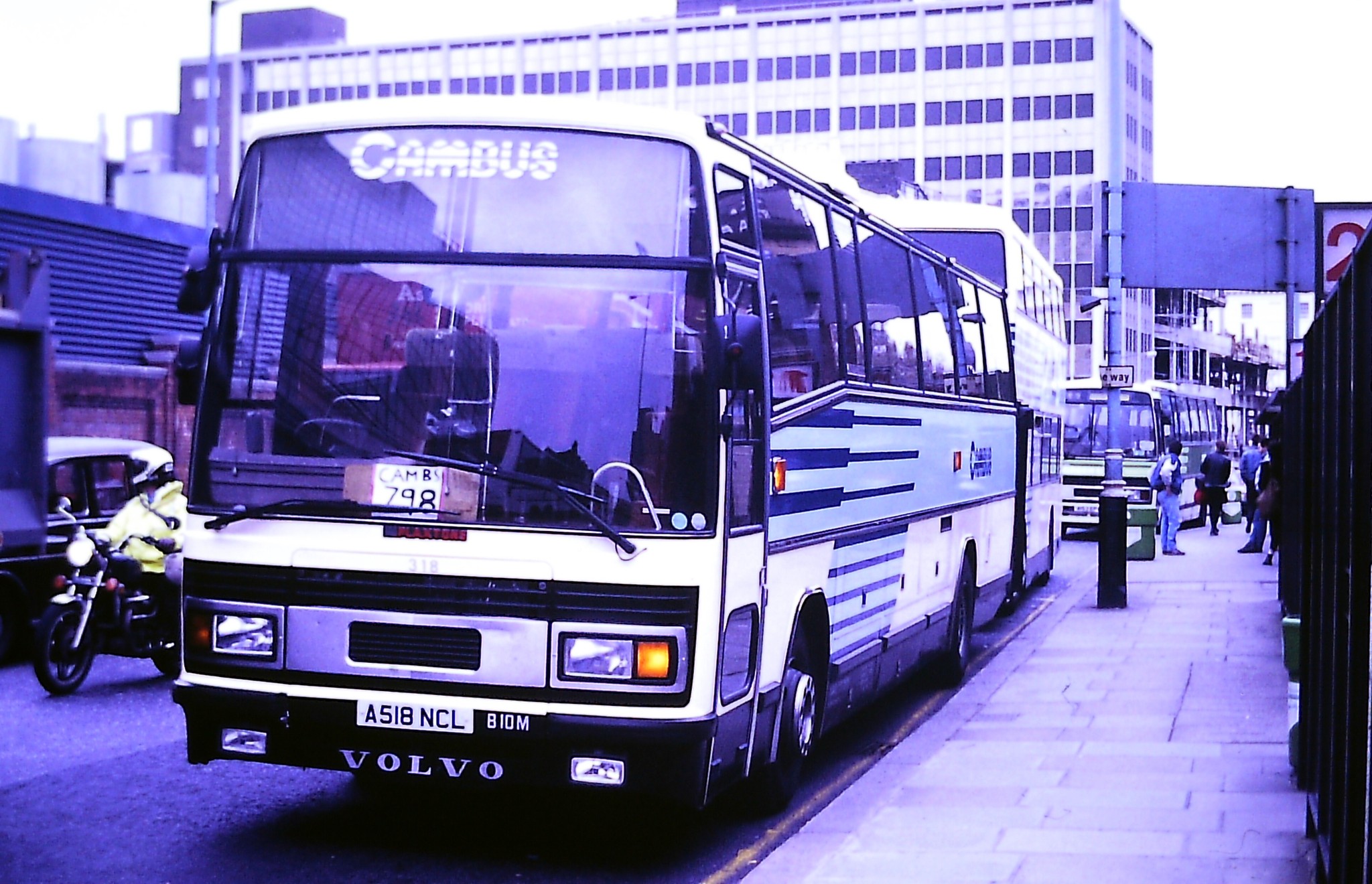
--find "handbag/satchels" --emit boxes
[1194,490,1207,504]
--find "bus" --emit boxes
[171,92,1069,828]
[1061,379,1222,540]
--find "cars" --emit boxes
[47,435,158,533]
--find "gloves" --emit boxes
[155,539,174,553]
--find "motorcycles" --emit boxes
[33,494,182,699]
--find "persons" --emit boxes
[1151,439,1185,555]
[1237,437,1283,566]
[1199,439,1231,536]
[1240,434,1262,532]
[95,448,189,574]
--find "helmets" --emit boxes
[129,447,174,486]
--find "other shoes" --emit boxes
[1162,548,1185,555]
[1246,520,1252,533]
[1263,554,1273,565]
[1237,542,1263,553]
[1210,529,1219,536]
